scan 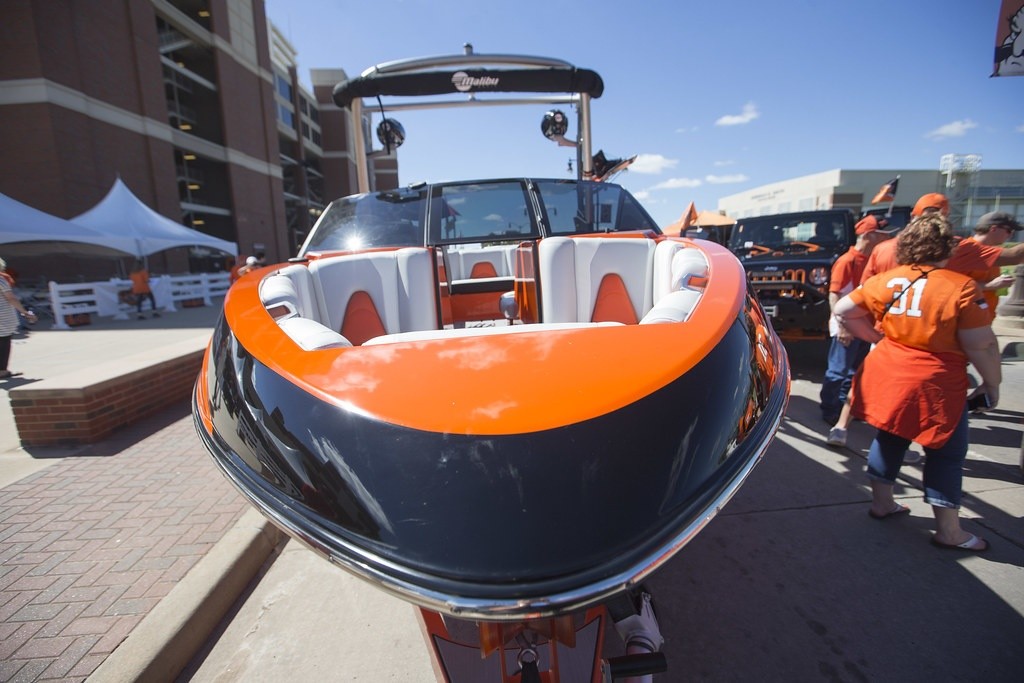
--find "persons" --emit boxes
[130,260,161,320]
[230,250,267,284]
[834,215,1003,554]
[0,257,39,380]
[819,192,987,464]
[943,211,1024,292]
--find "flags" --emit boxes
[871,178,897,206]
[681,201,698,231]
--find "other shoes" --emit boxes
[822,409,839,426]
[0,371,11,378]
[902,449,921,463]
[828,427,848,445]
[138,316,146,320]
[153,312,161,318]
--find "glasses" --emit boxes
[1000,226,1015,233]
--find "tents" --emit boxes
[0,176,245,279]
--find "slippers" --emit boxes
[868,503,911,518]
[930,531,991,551]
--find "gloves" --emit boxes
[20,312,37,324]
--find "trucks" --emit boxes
[727,202,916,350]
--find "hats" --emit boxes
[854,214,900,236]
[977,210,1024,231]
[913,193,950,218]
